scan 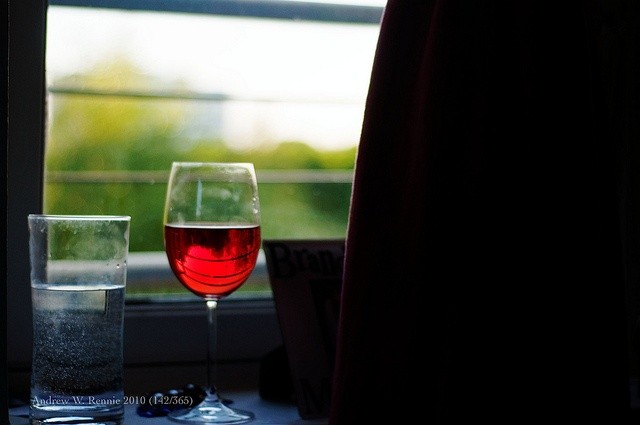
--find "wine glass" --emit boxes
[162,162,263,425]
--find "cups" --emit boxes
[27,214,131,425]
